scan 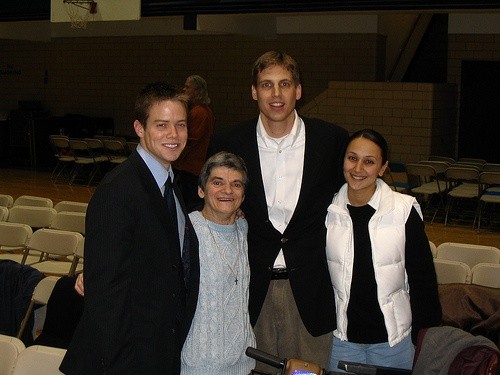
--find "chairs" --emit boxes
[429,240,500,289]
[0,194,87,375]
[49,135,138,187]
[378,154,500,232]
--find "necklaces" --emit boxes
[200,210,240,285]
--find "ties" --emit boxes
[165,176,186,290]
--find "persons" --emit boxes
[322,128,442,373]
[179,152,256,375]
[210,50,352,375]
[173,74,214,206]
[58,81,204,375]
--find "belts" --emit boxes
[271,269,290,280]
